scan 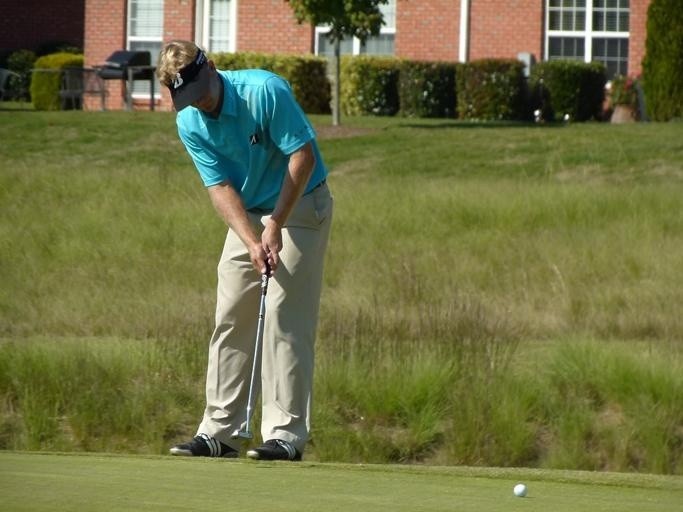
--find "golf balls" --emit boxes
[512,483,525,495]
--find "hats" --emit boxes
[164,48,210,112]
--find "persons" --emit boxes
[155,39,335,463]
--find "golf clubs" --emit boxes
[229,259,272,439]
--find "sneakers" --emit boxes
[170,433,239,457]
[248,439,301,460]
